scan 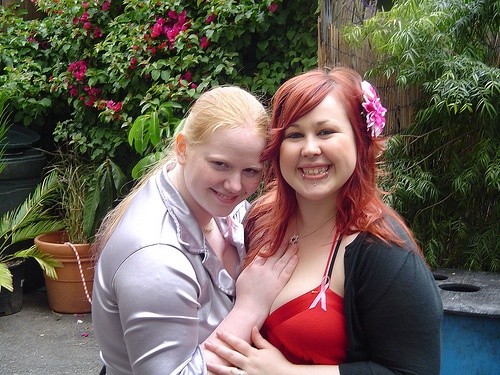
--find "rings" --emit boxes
[238,368,243,375]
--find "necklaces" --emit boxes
[200,218,215,233]
[289,210,339,244]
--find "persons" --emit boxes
[203,66,443,375]
[88,85,298,375]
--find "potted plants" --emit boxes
[36,138,99,313]
